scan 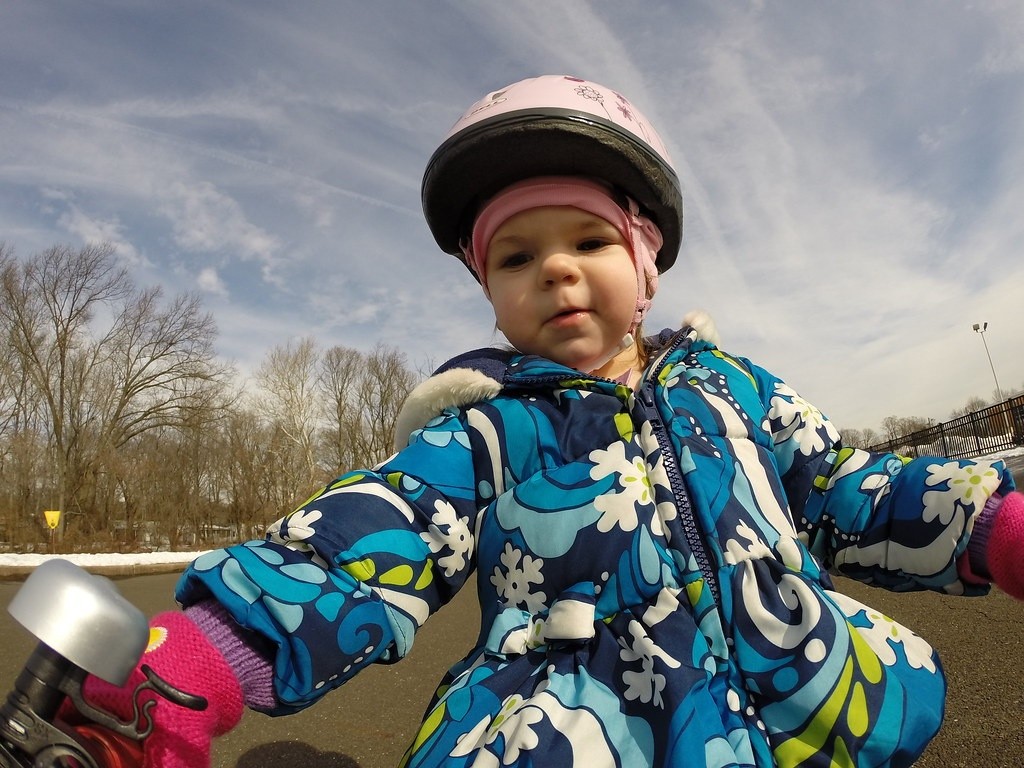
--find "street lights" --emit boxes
[973,321,1012,443]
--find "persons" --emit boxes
[61,74,1023,768]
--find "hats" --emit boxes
[470,174,663,296]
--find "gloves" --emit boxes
[56,601,281,768]
[967,492,1024,601]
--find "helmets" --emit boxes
[421,74,684,276]
